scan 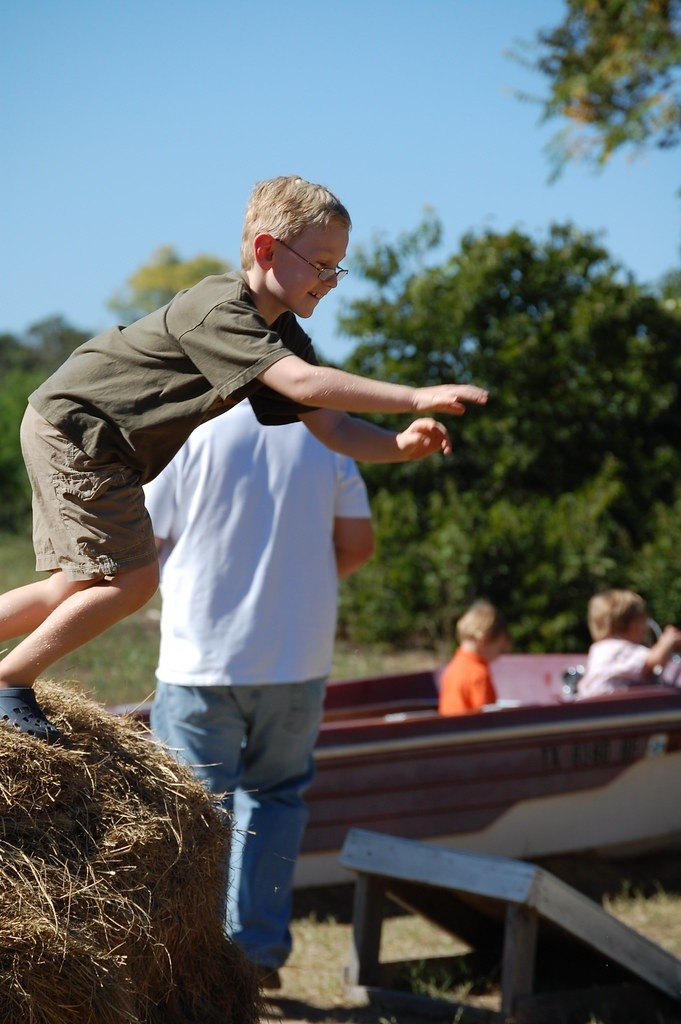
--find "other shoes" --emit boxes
[246,963,285,991]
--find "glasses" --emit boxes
[276,239,350,284]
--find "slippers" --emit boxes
[0,687,65,746]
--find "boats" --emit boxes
[113,651,681,892]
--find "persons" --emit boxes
[1,175,490,743]
[143,396,679,991]
